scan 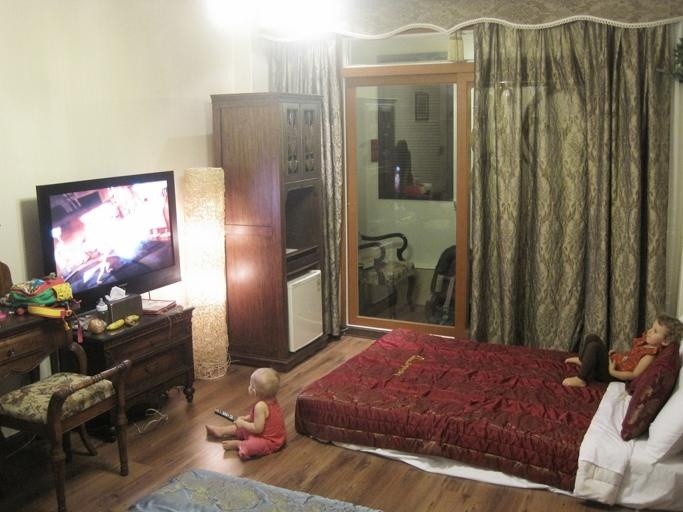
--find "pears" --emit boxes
[90,318,105,334]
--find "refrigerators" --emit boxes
[287,269,325,353]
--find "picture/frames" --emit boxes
[414,91,430,121]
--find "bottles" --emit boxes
[95,297,108,329]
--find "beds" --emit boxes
[295,324,682,511]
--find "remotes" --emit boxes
[215,408,238,422]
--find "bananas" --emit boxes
[125,314,140,327]
[106,319,125,330]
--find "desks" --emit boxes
[1,263,97,458]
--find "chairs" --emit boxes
[0,359,132,512]
[358,230,416,320]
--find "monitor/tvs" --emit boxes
[36,171,182,310]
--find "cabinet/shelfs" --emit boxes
[55,305,195,443]
[208,92,330,372]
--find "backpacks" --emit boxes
[0,274,73,318]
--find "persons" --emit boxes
[560,311,682,388]
[201,367,286,462]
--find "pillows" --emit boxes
[621,338,682,466]
